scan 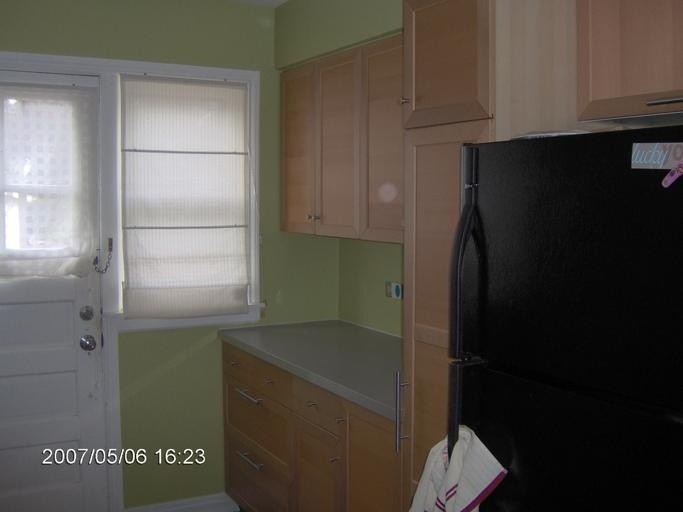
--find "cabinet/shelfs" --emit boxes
[394,120,492,511]
[398,0,578,131]
[573,1,683,125]
[219,342,403,512]
[362,34,407,243]
[278,46,361,238]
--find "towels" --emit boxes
[406,421,512,512]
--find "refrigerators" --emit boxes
[448,126,683,512]
